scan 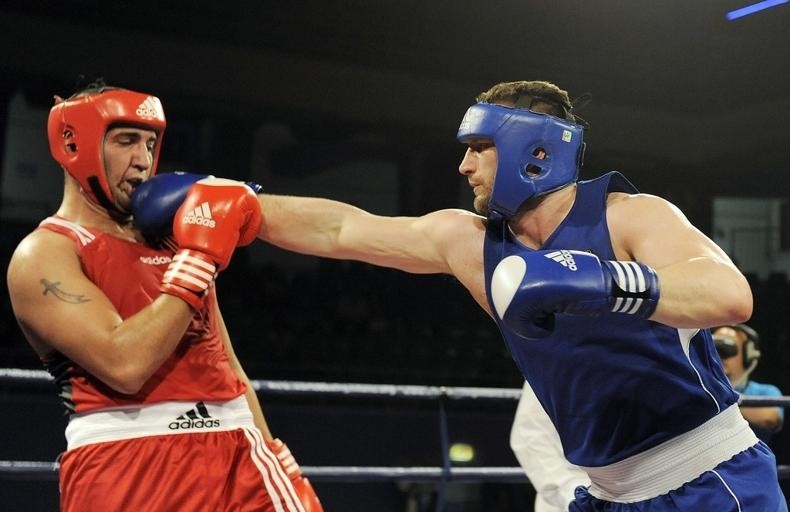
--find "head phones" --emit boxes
[710,323,761,370]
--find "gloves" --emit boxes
[132,169,263,247]
[158,177,262,315]
[266,437,324,512]
[490,249,660,342]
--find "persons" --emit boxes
[7,80,325,512]
[131,80,789,512]
[710,323,785,445]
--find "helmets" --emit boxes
[46,84,167,207]
[455,102,584,218]
